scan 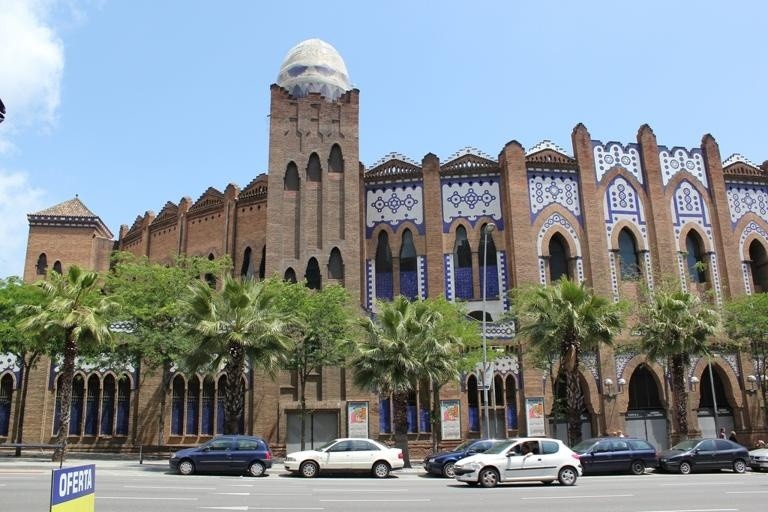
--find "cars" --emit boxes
[747,442,768,472]
[656,437,751,475]
[422,438,507,479]
[571,437,658,475]
[284,437,405,479]
[452,438,583,486]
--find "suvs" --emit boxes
[169,435,274,478]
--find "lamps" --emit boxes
[747,373,768,393]
[690,376,700,392]
[605,377,627,398]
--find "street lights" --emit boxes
[483,222,495,439]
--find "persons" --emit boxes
[602,430,626,448]
[720,426,726,439]
[729,431,737,442]
